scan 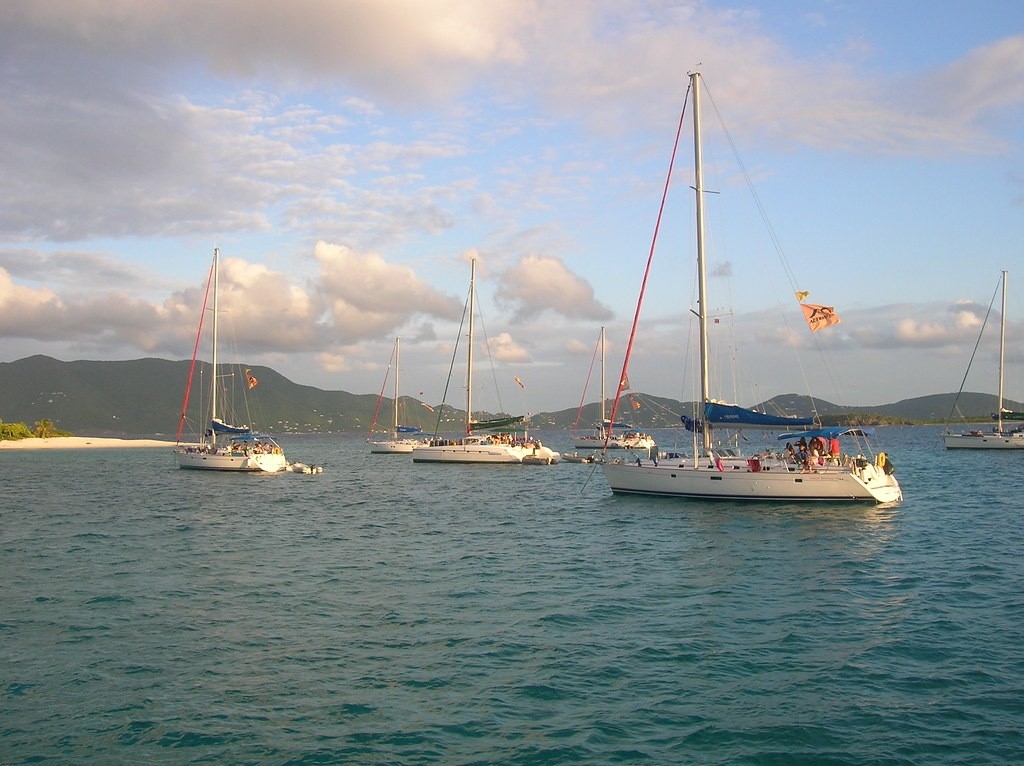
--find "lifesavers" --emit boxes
[717,459,724,471]
[878,451,885,467]
[646,435,651,442]
[274,447,280,455]
[493,438,499,446]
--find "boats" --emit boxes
[523,453,558,465]
[286,461,322,474]
[561,452,598,464]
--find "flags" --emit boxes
[801,303,839,334]
[629,397,641,410]
[205,429,218,437]
[620,375,630,391]
[796,290,808,301]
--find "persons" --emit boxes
[231,439,279,457]
[827,437,840,461]
[809,437,820,456]
[794,437,807,454]
[480,432,542,449]
[430,437,456,447]
[784,442,795,456]
[245,368,258,390]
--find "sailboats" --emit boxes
[600,71,904,504]
[366,336,418,454]
[173,247,287,473]
[572,327,658,449]
[413,258,561,464]
[940,270,1024,449]
